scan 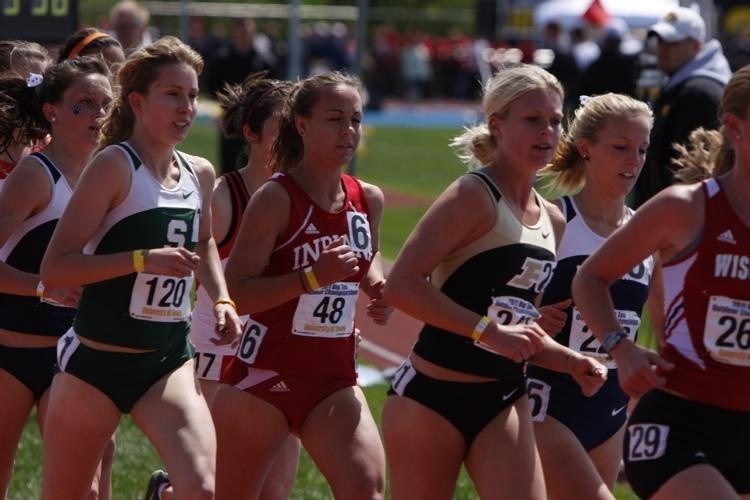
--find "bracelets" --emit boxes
[131,247,145,275]
[298,269,309,293]
[212,300,237,310]
[470,315,493,342]
[36,280,44,297]
[602,328,629,354]
[303,266,322,291]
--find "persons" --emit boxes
[571,58,750,500]
[38,35,245,499]
[211,71,398,499]
[49,0,749,209]
[380,60,608,499]
[2,54,117,499]
[0,25,130,190]
[525,90,667,499]
[141,78,300,500]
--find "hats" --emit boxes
[648,6,706,46]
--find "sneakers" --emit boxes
[142,468,169,500]
